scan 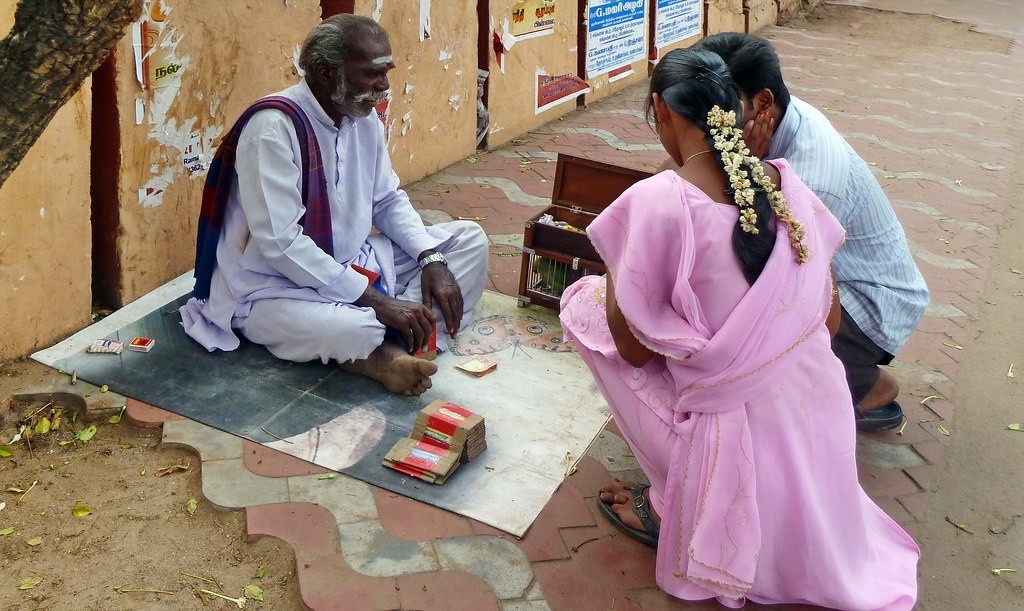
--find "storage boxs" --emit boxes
[517,152,654,310]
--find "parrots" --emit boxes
[532,257,583,294]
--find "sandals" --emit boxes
[597,482,662,548]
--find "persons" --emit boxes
[691,31,932,431]
[559,47,921,611]
[175,14,489,396]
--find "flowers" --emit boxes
[707,105,812,264]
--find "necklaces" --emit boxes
[684,150,713,166]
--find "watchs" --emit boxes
[418,252,448,270]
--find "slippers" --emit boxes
[855,400,902,430]
